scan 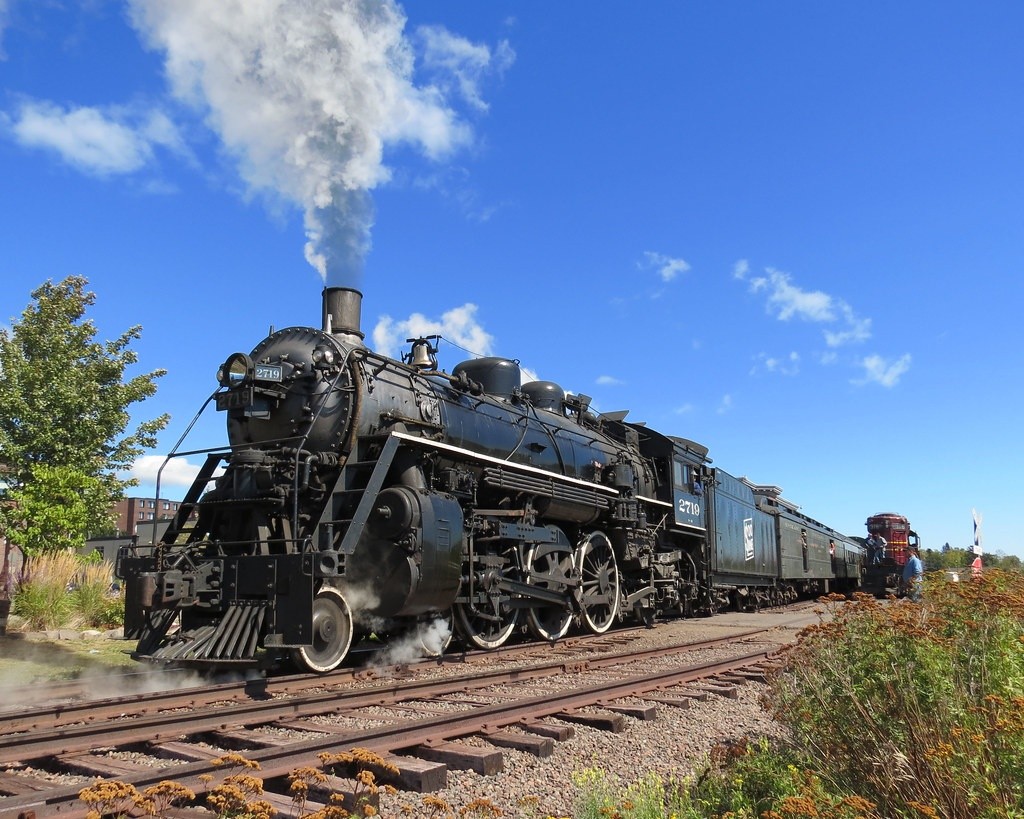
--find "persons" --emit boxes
[901,546,922,592]
[865,534,877,556]
[875,532,888,564]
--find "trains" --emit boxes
[114,286,921,682]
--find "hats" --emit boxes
[903,546,915,552]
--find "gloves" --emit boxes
[908,577,916,585]
